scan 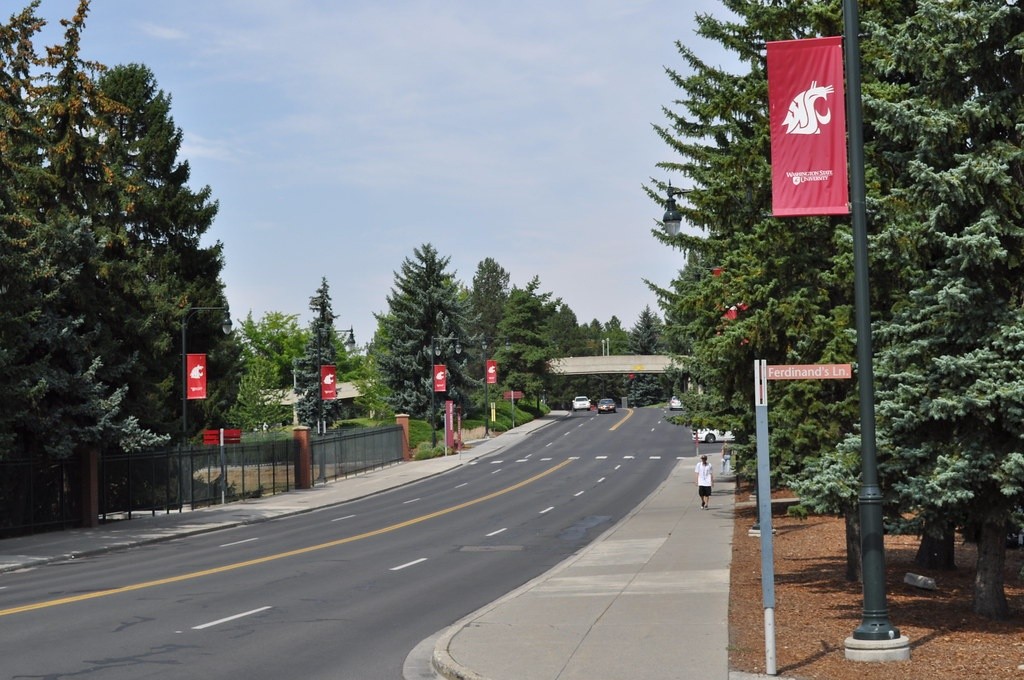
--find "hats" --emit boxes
[700,455,706,459]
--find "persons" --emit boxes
[719,440,731,476]
[695,454,715,510]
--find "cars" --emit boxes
[693,427,736,443]
[669,396,684,410]
[572,396,592,411]
[597,399,616,413]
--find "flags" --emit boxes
[713,267,751,345]
[765,36,850,216]
[186,353,207,400]
[487,360,497,383]
[321,365,337,400]
[434,365,446,392]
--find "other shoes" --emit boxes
[701,501,709,510]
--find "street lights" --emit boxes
[481,335,510,438]
[313,327,356,479]
[430,335,462,448]
[178,301,232,513]
